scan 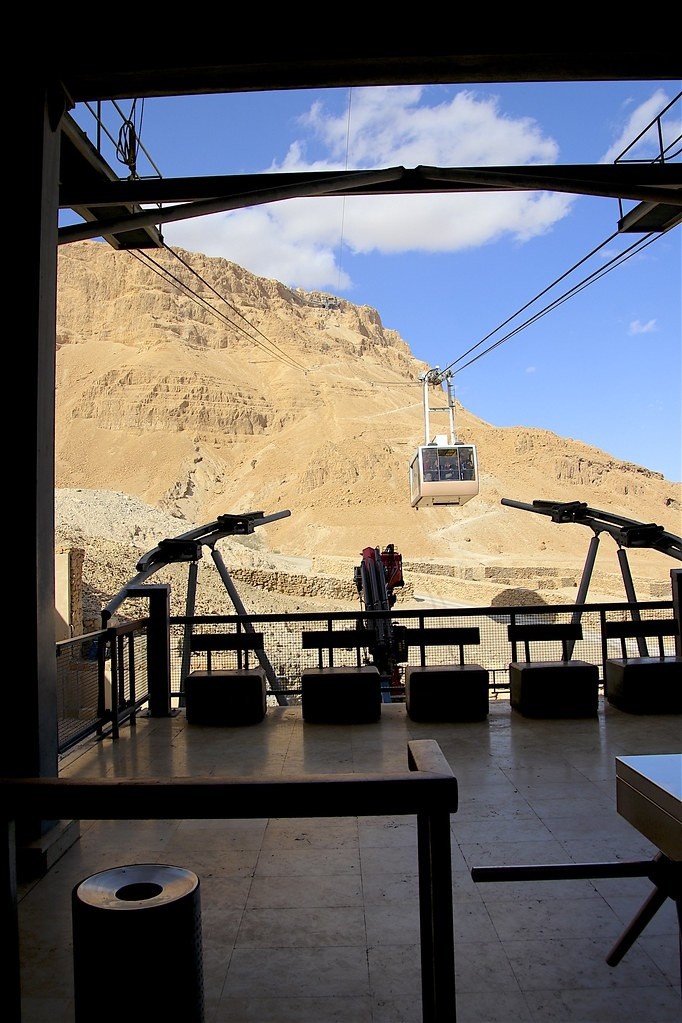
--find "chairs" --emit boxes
[303,630,382,723]
[605,619,682,716]
[182,634,266,725]
[507,624,599,719]
[402,626,490,723]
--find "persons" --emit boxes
[423,450,475,482]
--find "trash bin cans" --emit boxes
[71,861,202,1023]
